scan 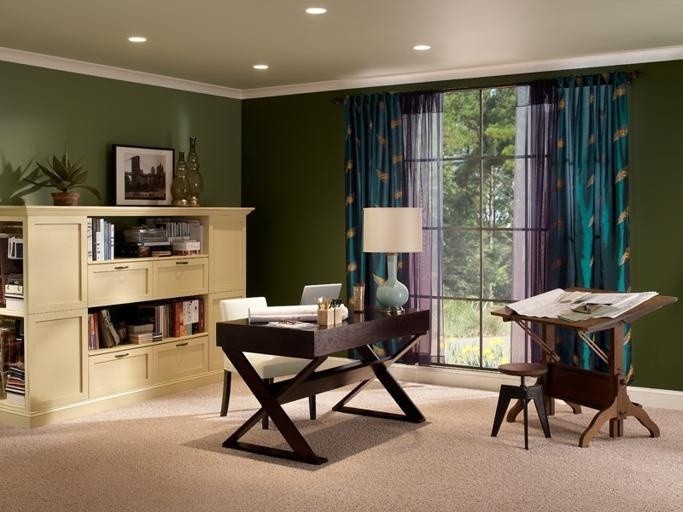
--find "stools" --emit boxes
[490,363,552,450]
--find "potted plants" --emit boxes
[21,149,103,205]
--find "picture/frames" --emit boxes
[112,143,176,207]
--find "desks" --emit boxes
[215,306,431,465]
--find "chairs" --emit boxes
[217,294,316,429]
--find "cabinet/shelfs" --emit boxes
[0,206,256,430]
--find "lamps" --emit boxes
[361,206,423,314]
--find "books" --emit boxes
[88,299,170,350]
[4,367,24,395]
[156,220,190,236]
[87,217,115,261]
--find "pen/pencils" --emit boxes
[313,296,342,309]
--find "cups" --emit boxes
[353,286,366,313]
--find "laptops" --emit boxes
[298,282,342,305]
